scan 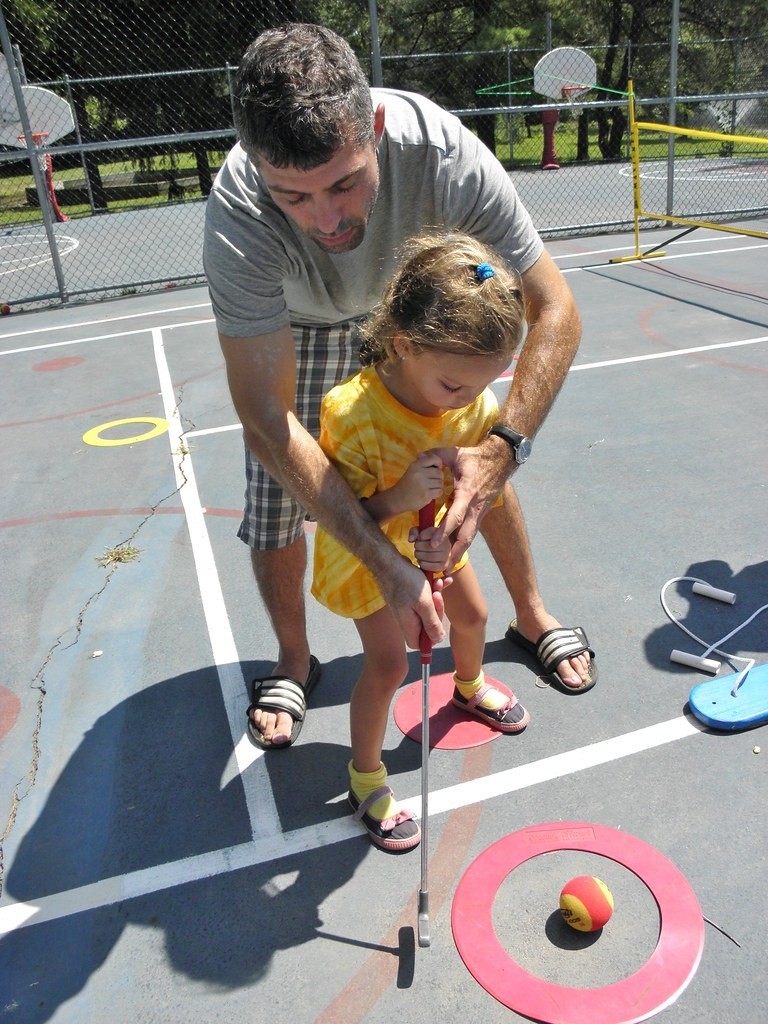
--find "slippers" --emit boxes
[508,614,598,695]
[246,655,321,748]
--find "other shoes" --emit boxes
[348,787,421,850]
[451,682,530,732]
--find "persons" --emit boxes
[203,22,599,751]
[310,232,530,851]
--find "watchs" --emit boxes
[486,424,532,464]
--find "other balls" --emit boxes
[560,876,615,932]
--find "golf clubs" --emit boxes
[412,457,440,948]
